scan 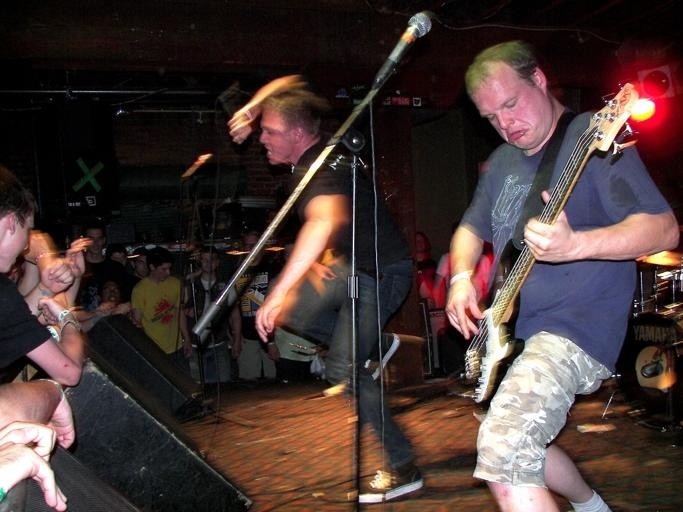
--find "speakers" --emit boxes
[86,314,195,415]
[30,345,254,512]
[0,440,138,512]
[428,309,452,368]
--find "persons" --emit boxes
[227,74,426,504]
[0,161,92,512]
[442,36,679,512]
[431,219,491,379]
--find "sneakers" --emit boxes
[358,463,426,503]
[370,333,400,379]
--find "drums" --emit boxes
[616,301,683,426]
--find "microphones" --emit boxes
[641,363,663,378]
[373,11,433,88]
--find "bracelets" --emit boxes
[240,108,253,122]
[447,270,472,288]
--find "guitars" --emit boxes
[461,62,640,404]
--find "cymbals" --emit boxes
[634,251,683,266]
